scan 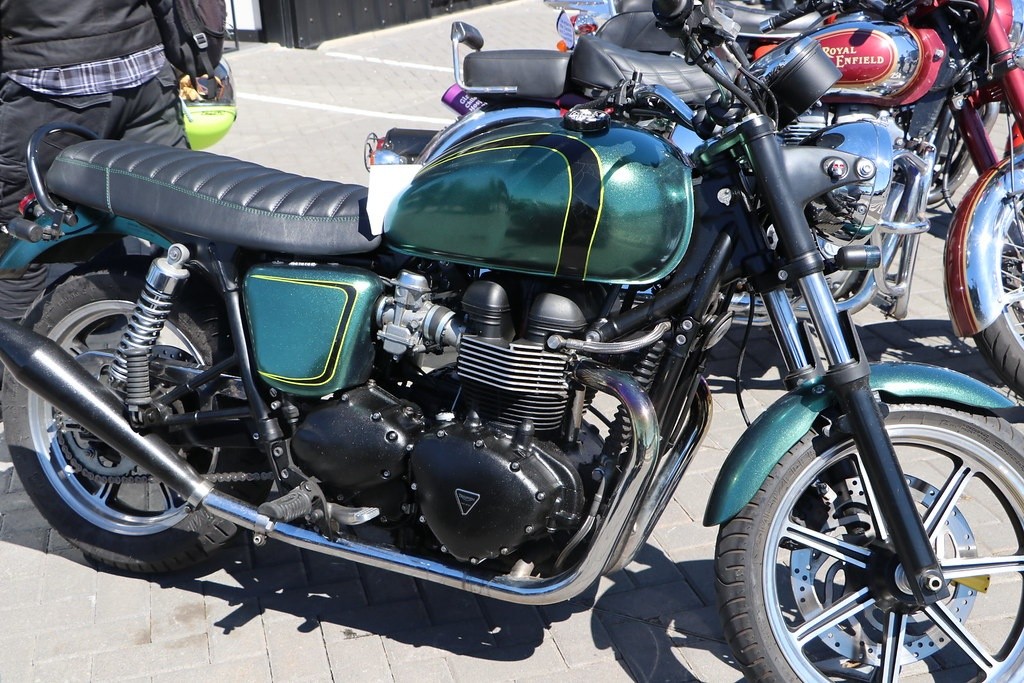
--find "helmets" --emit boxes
[181,61,237,149]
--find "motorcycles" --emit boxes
[2,2,1024,683]
[359,2,1024,380]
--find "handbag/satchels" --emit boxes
[174,0,227,80]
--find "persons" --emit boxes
[0,0,191,324]
